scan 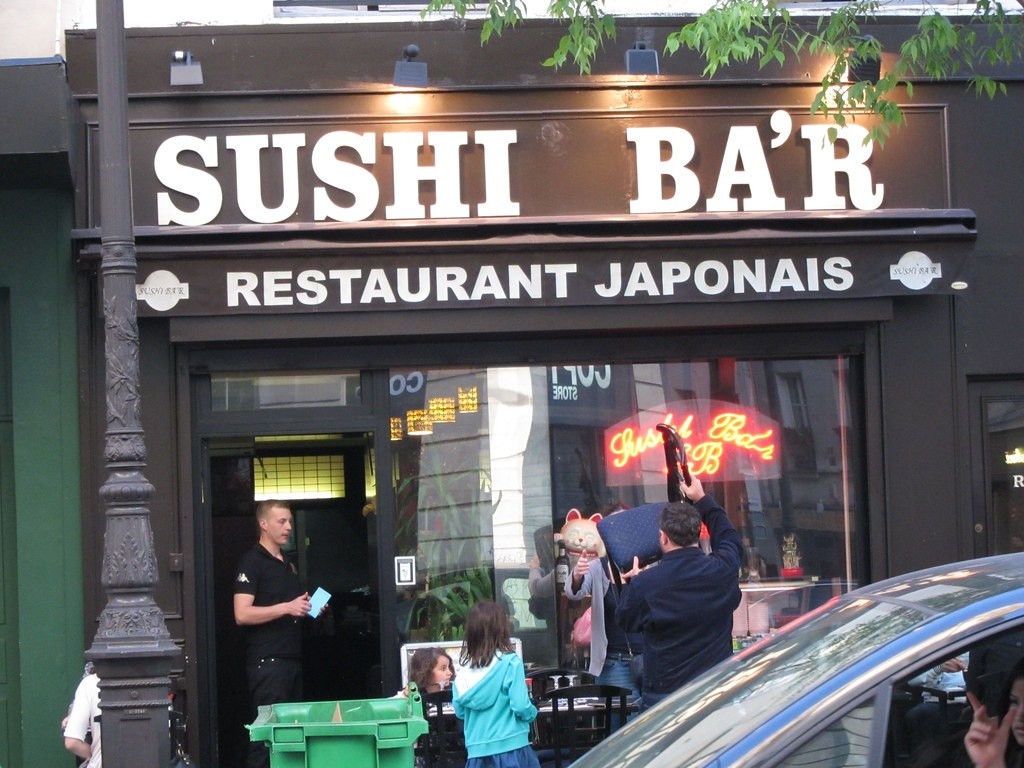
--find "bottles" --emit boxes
[555,540,571,593]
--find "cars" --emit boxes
[564,551,1024,767]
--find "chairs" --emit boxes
[420,666,632,768]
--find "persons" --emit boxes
[392,646,457,700]
[963,666,1024,768]
[527,552,555,658]
[907,652,969,723]
[61,660,111,768]
[233,498,329,768]
[615,475,743,714]
[564,503,648,733]
[452,600,540,768]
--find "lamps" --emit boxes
[429,397,456,422]
[170,50,204,86]
[407,409,433,435]
[845,35,882,82]
[458,385,480,413]
[391,43,428,88]
[626,40,658,74]
[390,418,403,440]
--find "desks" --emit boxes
[738,582,816,636]
[426,700,641,751]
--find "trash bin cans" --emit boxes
[243,681,430,768]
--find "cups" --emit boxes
[746,546,760,580]
